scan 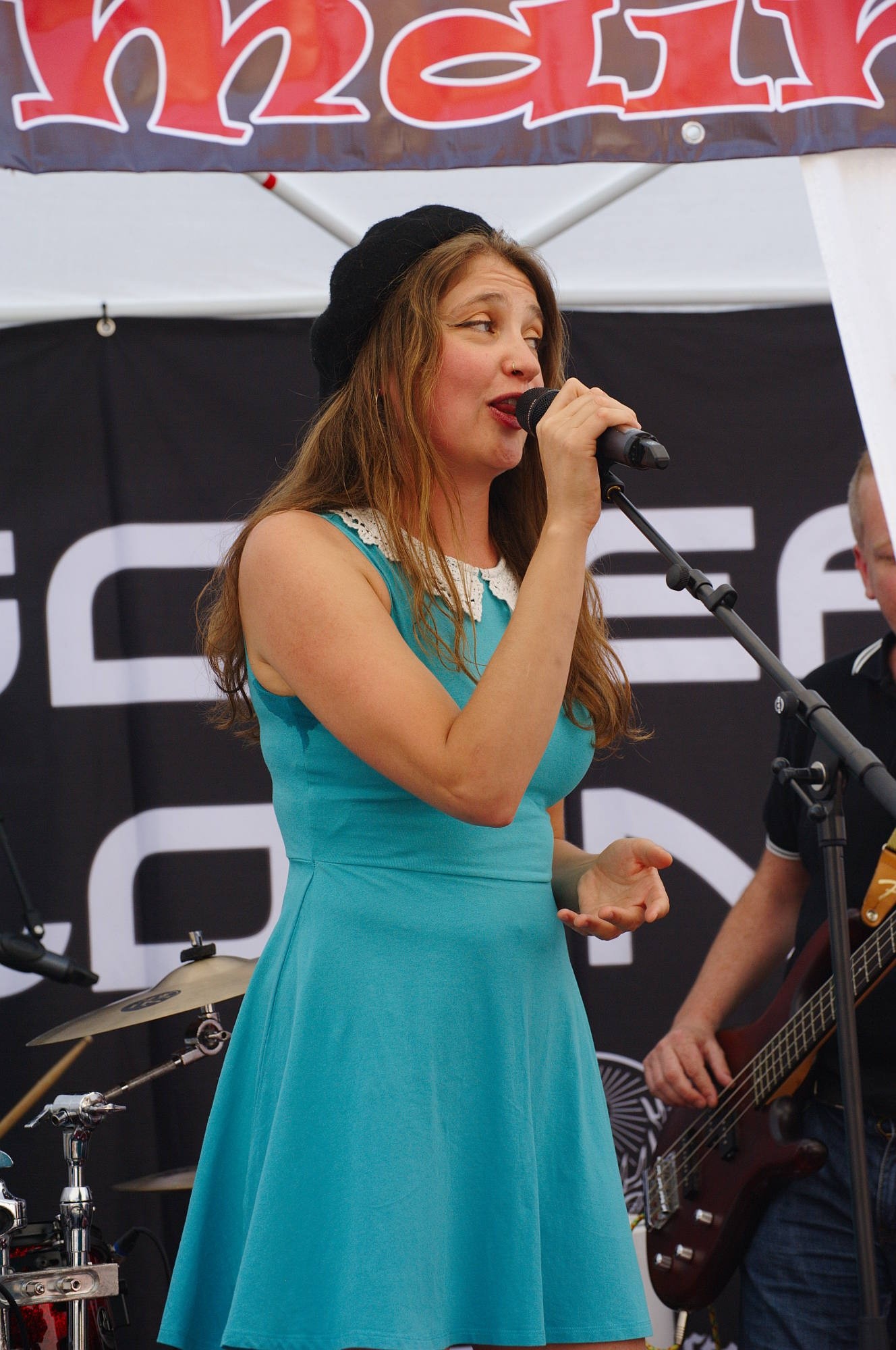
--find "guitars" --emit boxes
[643,827,896,1313]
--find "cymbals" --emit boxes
[24,950,266,1051]
[112,1165,198,1191]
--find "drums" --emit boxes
[0,1220,120,1349]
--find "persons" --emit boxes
[156,205,672,1350]
[641,452,896,1350]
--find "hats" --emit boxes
[308,203,495,402]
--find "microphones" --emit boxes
[0,925,101,987]
[109,1230,140,1264]
[516,387,669,471]
